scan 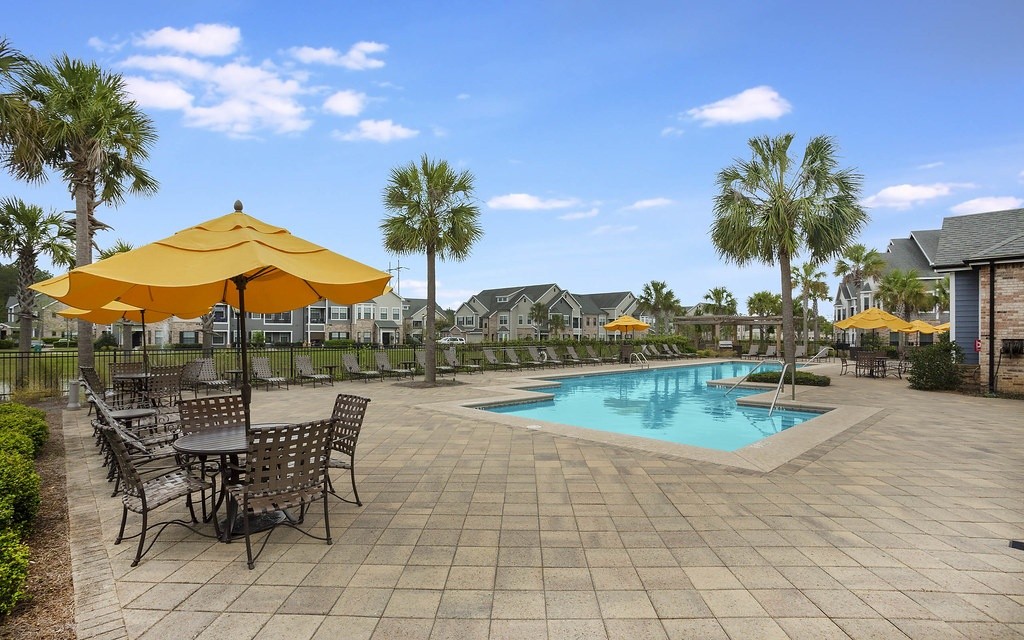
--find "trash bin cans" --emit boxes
[838,344,850,357]
[621,340,634,364]
[732,345,742,357]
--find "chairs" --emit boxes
[837,348,909,379]
[740,345,830,362]
[641,344,700,361]
[78,345,618,570]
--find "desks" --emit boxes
[401,361,416,378]
[859,356,890,378]
[108,409,156,431]
[470,358,484,374]
[115,372,178,407]
[323,365,339,383]
[225,370,243,391]
[173,423,317,543]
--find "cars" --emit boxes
[435,336,466,345]
